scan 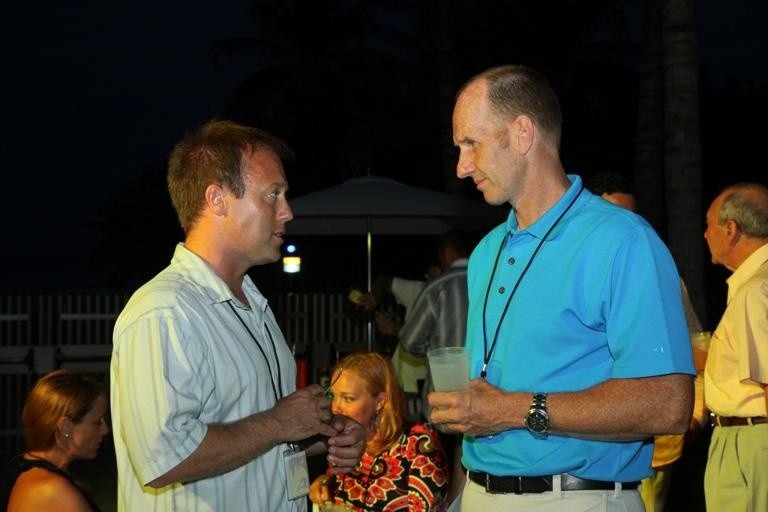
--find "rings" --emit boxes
[319,479,328,486]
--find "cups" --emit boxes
[690,332,713,374]
[427,347,472,433]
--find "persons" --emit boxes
[425,65,696,511]
[592,181,703,512]
[401,229,478,493]
[355,291,406,347]
[309,352,455,512]
[0,363,121,512]
[699,181,768,511]
[360,252,443,325]
[109,121,369,512]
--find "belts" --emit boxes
[462,466,641,494]
[709,412,768,426]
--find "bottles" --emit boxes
[347,288,379,315]
[317,359,330,391]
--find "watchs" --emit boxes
[522,390,550,441]
